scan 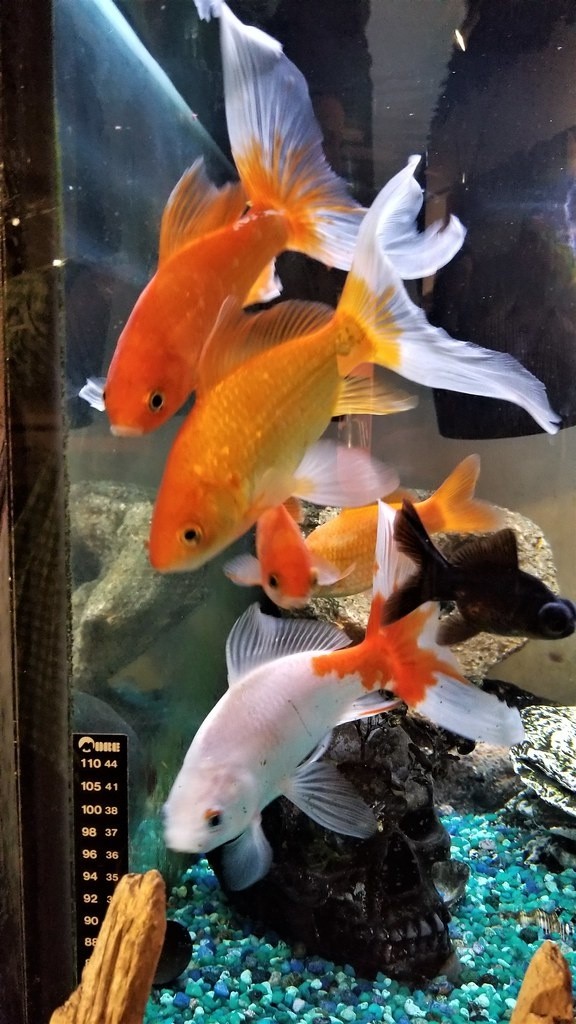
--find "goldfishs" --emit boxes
[79,0,565,572]
[160,453,576,891]
